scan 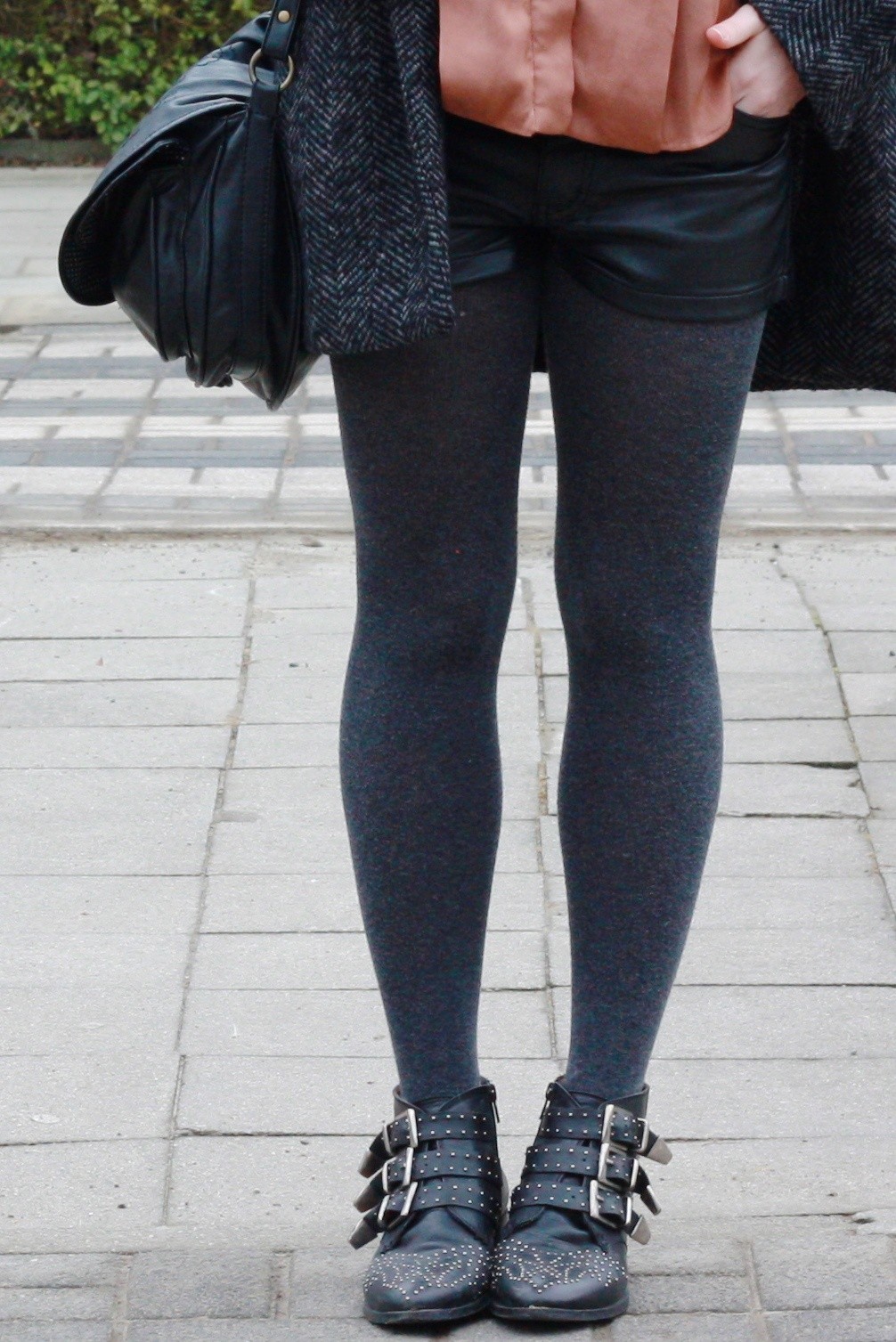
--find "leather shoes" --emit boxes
[493,1077,649,1323]
[363,1078,492,1326]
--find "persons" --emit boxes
[259,1,896,1328]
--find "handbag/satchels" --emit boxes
[57,8,326,411]
